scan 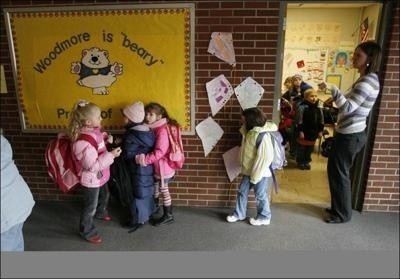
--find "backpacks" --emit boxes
[154,119,185,171]
[321,135,333,158]
[255,131,286,172]
[45,130,99,193]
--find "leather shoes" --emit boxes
[81,231,102,244]
[98,215,112,220]
[327,217,335,223]
[327,207,333,212]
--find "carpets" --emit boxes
[22,200,400,251]
[271,127,333,203]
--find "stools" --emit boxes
[318,123,335,155]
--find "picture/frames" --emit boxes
[3,3,195,135]
[325,74,342,94]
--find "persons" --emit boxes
[71,101,122,245]
[0,126,35,251]
[227,108,277,226]
[106,101,158,233]
[291,75,303,122]
[281,76,295,145]
[299,83,313,162]
[296,89,324,170]
[318,38,382,225]
[134,102,176,228]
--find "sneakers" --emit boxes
[249,216,270,225]
[227,214,246,222]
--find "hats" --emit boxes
[300,81,312,96]
[124,102,144,123]
[292,74,302,79]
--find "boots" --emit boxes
[153,198,161,214]
[156,205,174,226]
